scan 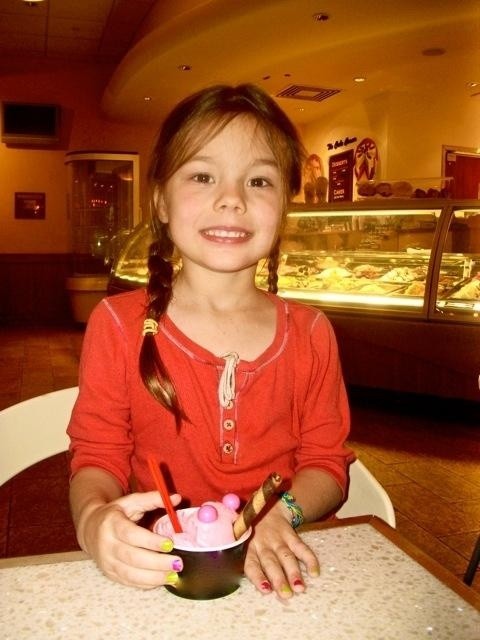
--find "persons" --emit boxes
[65,82,357,602]
[303,150,328,202]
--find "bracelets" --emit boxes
[279,492,305,528]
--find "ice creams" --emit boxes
[150,472,281,552]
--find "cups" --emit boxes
[151,505,254,601]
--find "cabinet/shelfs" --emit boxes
[105,199,480,413]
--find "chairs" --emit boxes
[0,384,396,541]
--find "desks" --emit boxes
[0,514,478,640]
